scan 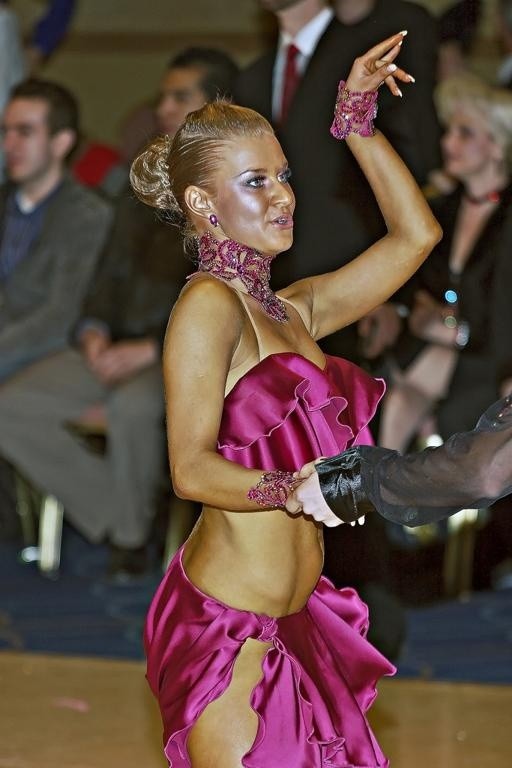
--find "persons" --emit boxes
[145,28,446,768]
[2,73,106,385]
[226,0,511,365]
[343,72,512,607]
[2,40,247,590]
[283,391,512,528]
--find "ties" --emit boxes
[281,45,298,121]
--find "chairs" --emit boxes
[1,398,480,599]
